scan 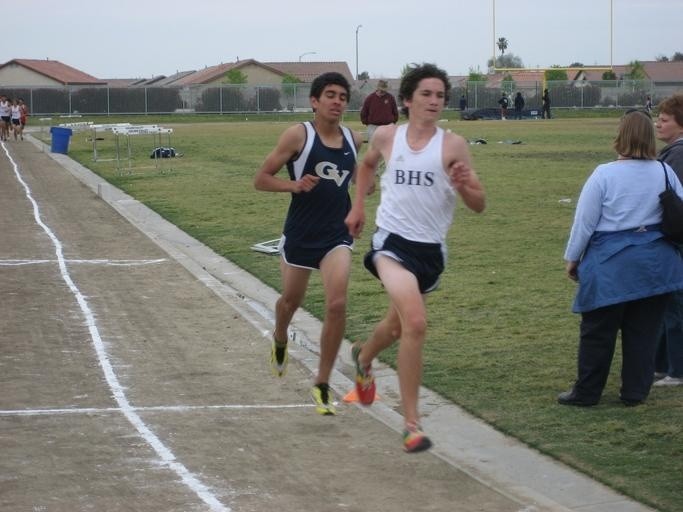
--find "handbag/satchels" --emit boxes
[656,159,683,254]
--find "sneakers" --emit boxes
[310,382,337,417]
[400,421,432,456]
[269,326,291,377]
[557,389,600,407]
[654,373,683,389]
[349,343,376,409]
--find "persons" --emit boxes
[514,92,525,120]
[651,94,683,387]
[0,95,27,142]
[360,79,398,176]
[254,71,376,416]
[459,95,468,121]
[498,93,509,121]
[645,96,654,113]
[343,63,486,452]
[541,89,552,120]
[557,109,683,407]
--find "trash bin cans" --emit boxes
[51,127,72,153]
